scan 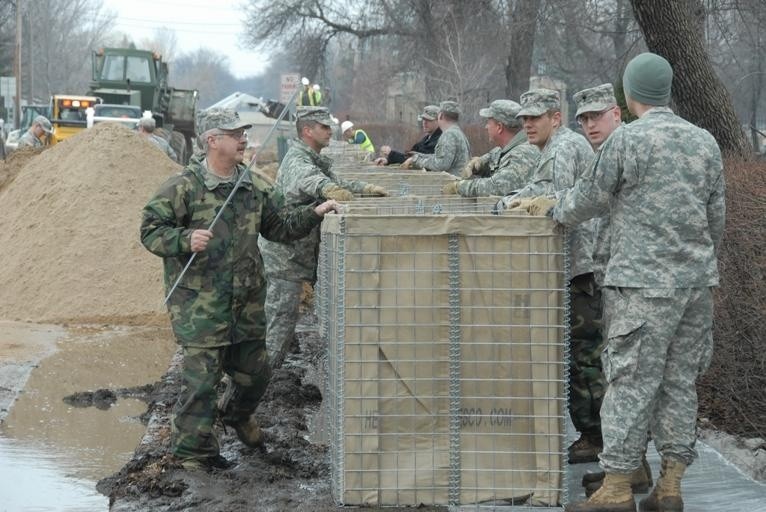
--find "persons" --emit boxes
[493,86,607,461]
[340,121,375,153]
[299,78,331,110]
[219,106,387,385]
[136,118,176,161]
[375,105,442,166]
[573,81,655,496]
[18,115,52,150]
[524,54,726,510]
[443,99,538,197]
[462,145,501,180]
[401,101,470,176]
[141,109,341,473]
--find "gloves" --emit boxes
[527,197,556,216]
[464,156,483,177]
[508,200,529,212]
[324,186,354,202]
[363,185,389,197]
[442,182,459,195]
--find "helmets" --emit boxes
[302,78,310,85]
[342,122,354,134]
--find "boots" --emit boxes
[568,436,686,512]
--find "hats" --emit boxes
[196,107,252,134]
[623,53,672,105]
[573,83,615,119]
[295,105,336,126]
[439,102,462,115]
[479,100,522,129]
[136,118,156,131]
[514,89,560,118]
[35,114,52,134]
[420,106,439,121]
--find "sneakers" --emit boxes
[234,416,265,447]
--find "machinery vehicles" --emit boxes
[19,45,199,168]
